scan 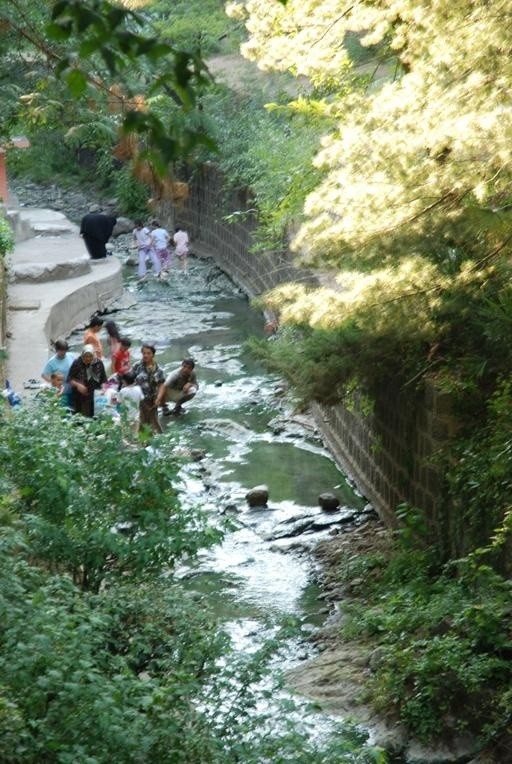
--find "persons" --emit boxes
[133,219,140,229]
[132,222,162,278]
[172,225,189,274]
[40,316,199,443]
[151,220,172,266]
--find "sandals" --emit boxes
[162,407,185,416]
